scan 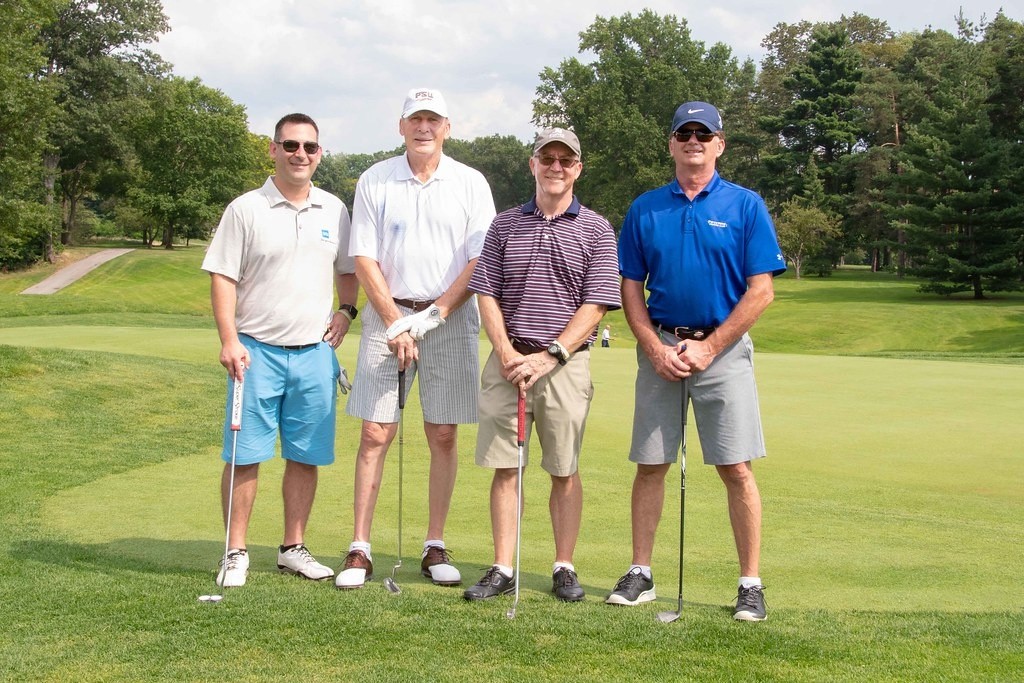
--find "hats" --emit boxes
[401,88,449,118]
[534,127,581,156]
[671,102,723,132]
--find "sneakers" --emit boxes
[276,545,334,580]
[420,544,461,584]
[606,567,656,604]
[463,565,516,599]
[216,549,249,587]
[730,585,769,622]
[551,567,585,601]
[336,550,373,588]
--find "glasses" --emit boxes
[673,128,718,142]
[532,155,579,168]
[275,140,320,154]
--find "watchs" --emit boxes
[339,304,358,319]
[546,343,567,366]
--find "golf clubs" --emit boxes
[383,368,405,593]
[506,387,524,619]
[656,343,688,624]
[198,357,245,601]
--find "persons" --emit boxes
[201,114,360,588]
[464,128,622,602]
[606,101,790,621]
[334,87,497,590]
[602,325,610,347]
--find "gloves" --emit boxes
[385,305,446,340]
[337,367,352,395]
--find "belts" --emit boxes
[647,319,719,342]
[393,296,435,312]
[510,340,588,357]
[274,344,317,351]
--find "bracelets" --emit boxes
[552,340,570,360]
[337,310,352,324]
